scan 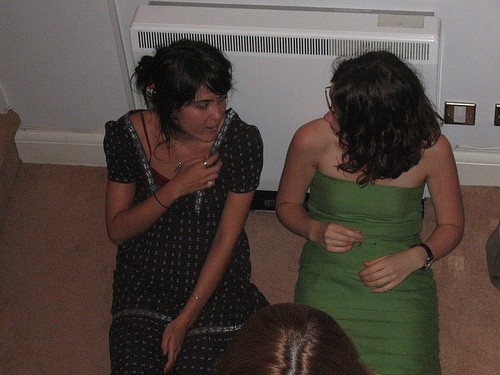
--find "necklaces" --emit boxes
[171,137,193,168]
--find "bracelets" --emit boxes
[154,192,169,208]
[190,294,204,310]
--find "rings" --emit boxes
[203,160,210,168]
[207,180,213,188]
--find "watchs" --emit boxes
[411,243,435,271]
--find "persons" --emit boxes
[103,40,270,375]
[276,51,465,375]
[215,303,374,375]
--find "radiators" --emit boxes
[130,3,443,192]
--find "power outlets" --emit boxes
[444,102,476,126]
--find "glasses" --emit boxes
[325,86,334,114]
